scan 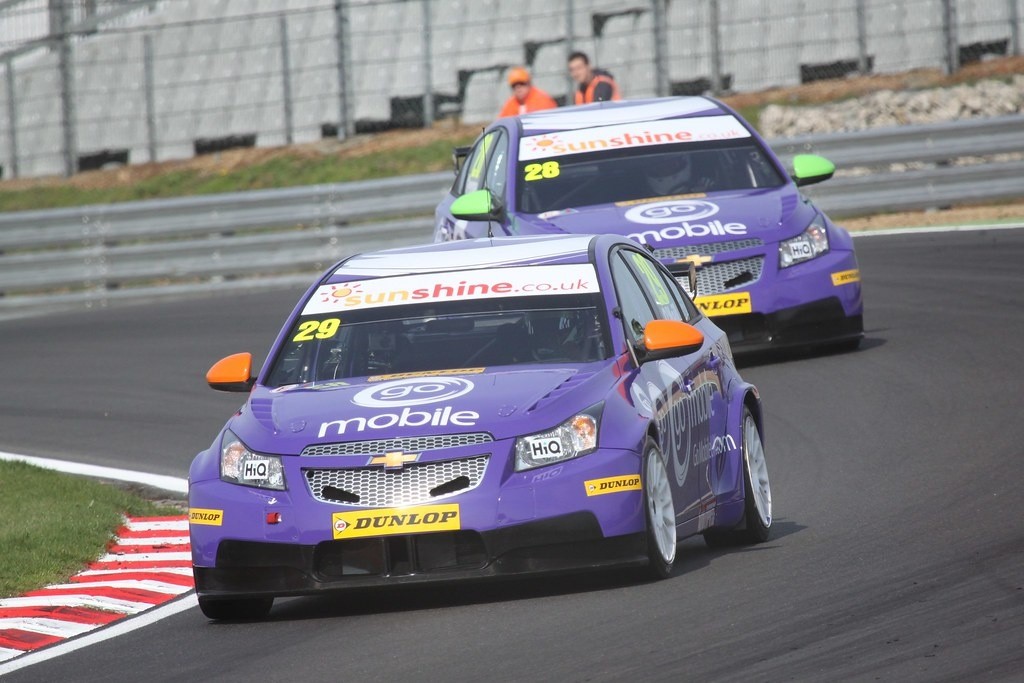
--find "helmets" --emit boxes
[639,150,693,197]
[524,304,583,363]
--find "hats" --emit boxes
[508,68,529,86]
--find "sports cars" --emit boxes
[185,230,774,622]
[433,94,865,352]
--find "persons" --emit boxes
[498,68,558,120]
[648,153,714,197]
[568,52,620,104]
[496,306,603,365]
